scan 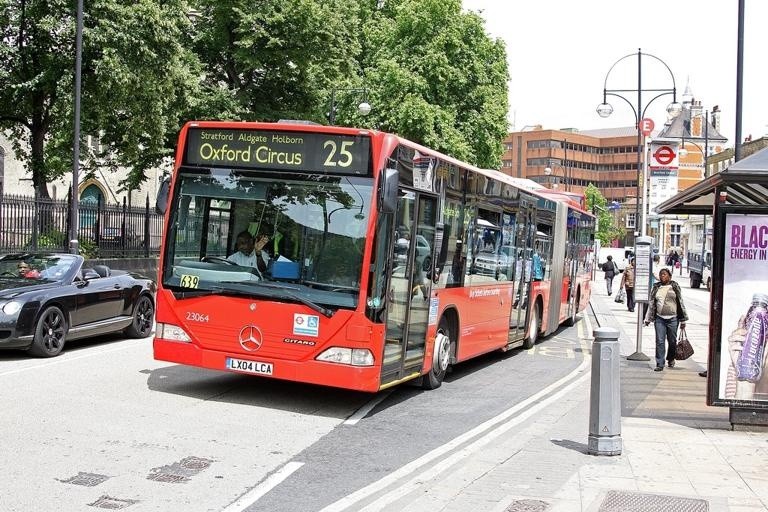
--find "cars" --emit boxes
[412,232,548,287]
[49,222,148,258]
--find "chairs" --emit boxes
[82,264,112,280]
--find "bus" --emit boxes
[151,117,600,396]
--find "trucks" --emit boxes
[686,246,714,292]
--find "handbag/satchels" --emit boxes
[614,268,619,275]
[615,288,625,304]
[672,340,694,360]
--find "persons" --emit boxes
[602,255,619,296]
[615,255,636,312]
[15,259,40,281]
[395,248,429,326]
[221,229,271,282]
[642,267,688,371]
[667,250,683,265]
[513,253,595,282]
[724,302,768,399]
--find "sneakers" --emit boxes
[668,360,675,367]
[699,371,707,376]
[654,366,663,371]
[628,308,634,312]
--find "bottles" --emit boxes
[735,293,768,382]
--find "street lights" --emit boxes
[679,104,724,178]
[324,84,373,127]
[542,158,569,192]
[591,48,684,362]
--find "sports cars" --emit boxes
[0,251,156,358]
